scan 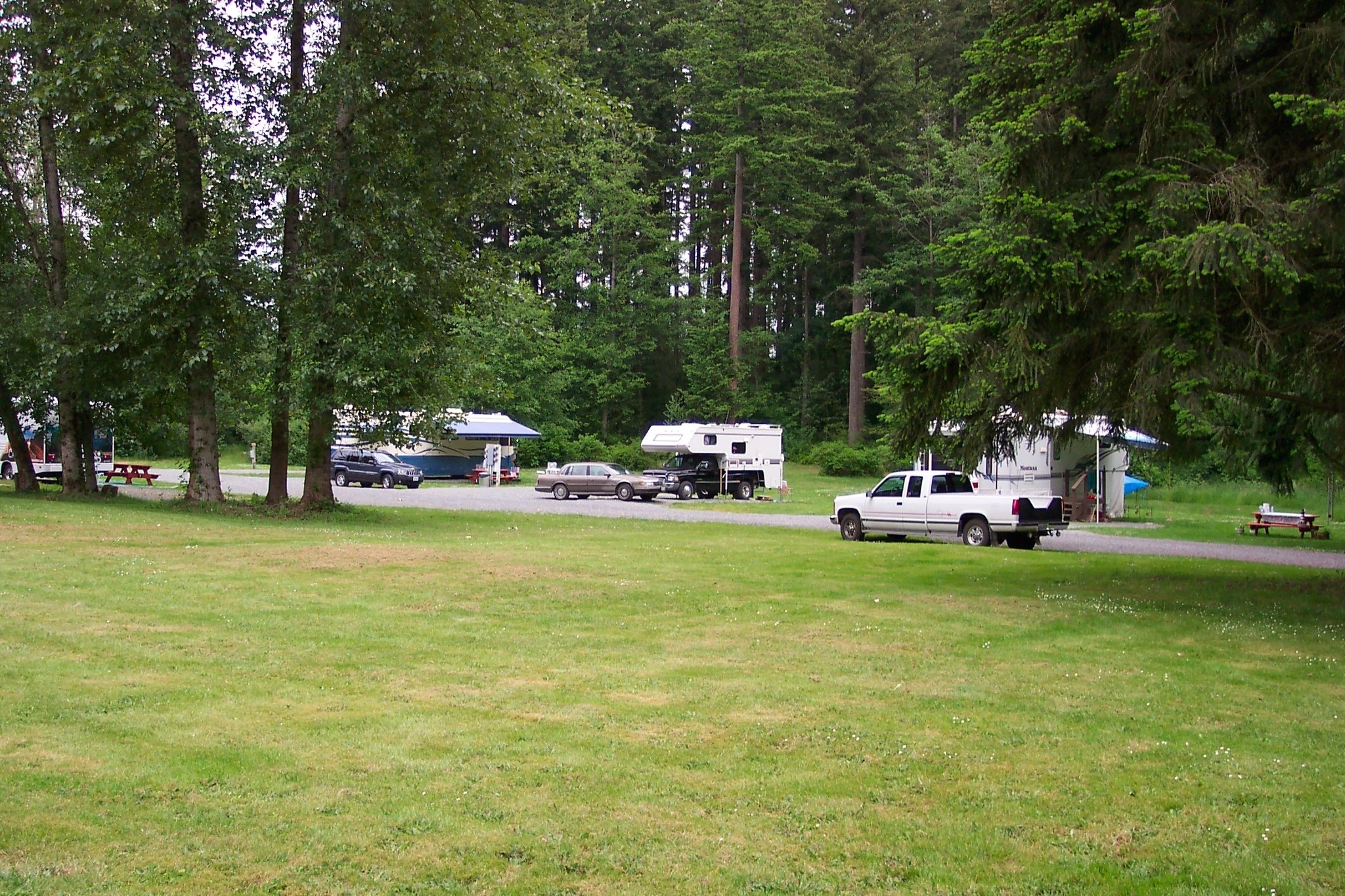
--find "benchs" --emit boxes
[104,472,159,479]
[466,475,513,479]
[1245,522,1324,528]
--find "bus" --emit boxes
[0,392,119,482]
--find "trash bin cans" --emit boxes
[478,472,490,487]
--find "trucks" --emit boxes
[640,420,785,501]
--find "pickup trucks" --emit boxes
[828,468,1070,548]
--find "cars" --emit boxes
[533,460,667,502]
[330,447,425,490]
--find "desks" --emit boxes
[1251,511,1320,538]
[105,464,152,486]
[472,469,509,484]
